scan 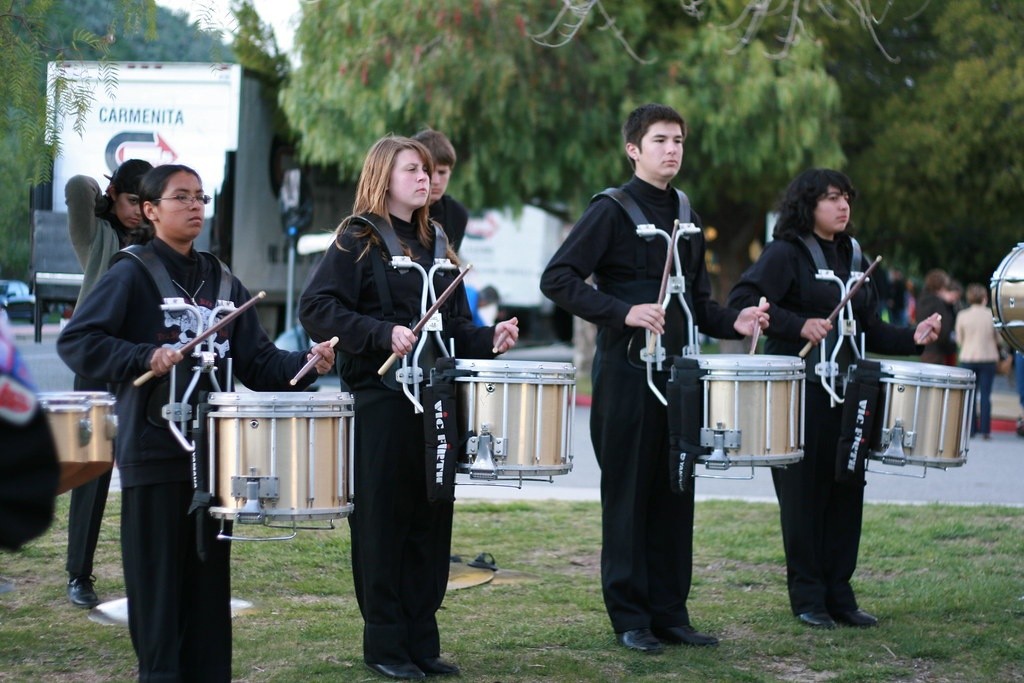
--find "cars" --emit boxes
[0,280,35,324]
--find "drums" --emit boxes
[989,243,1024,354]
[453,356,578,478]
[35,386,118,464]
[206,389,355,523]
[686,353,808,470]
[866,353,978,470]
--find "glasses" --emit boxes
[155,193,212,205]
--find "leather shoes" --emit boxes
[799,611,837,629]
[829,608,878,626]
[66,574,101,608]
[412,657,459,676]
[615,628,663,652]
[365,660,425,681]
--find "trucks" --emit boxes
[455,199,576,347]
[27,60,347,341]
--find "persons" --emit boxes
[298,129,519,679]
[914,268,1003,438]
[56,159,336,683]
[538,104,769,657]
[0,316,58,553]
[724,168,943,628]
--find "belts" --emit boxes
[652,624,718,647]
